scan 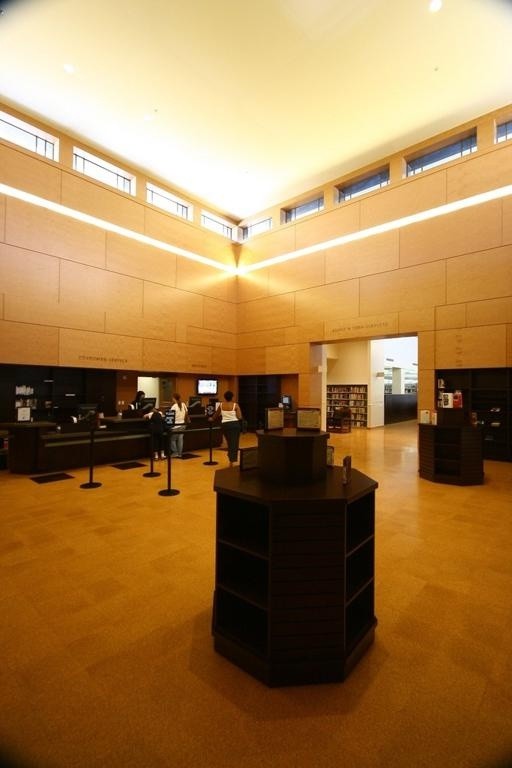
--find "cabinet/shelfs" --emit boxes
[419,367,512,486]
[0,363,87,424]
[326,384,368,427]
[210,425,379,688]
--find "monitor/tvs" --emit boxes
[141,398,156,411]
[197,378,218,395]
[189,396,202,407]
[281,395,291,405]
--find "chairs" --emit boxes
[328,407,352,433]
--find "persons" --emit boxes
[169,391,193,459]
[142,405,168,461]
[207,390,242,470]
[127,390,146,414]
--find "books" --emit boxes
[418,378,463,426]
[486,405,504,416]
[487,419,503,432]
[327,385,367,429]
[484,431,496,442]
[471,412,478,425]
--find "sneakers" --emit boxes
[154,454,182,461]
